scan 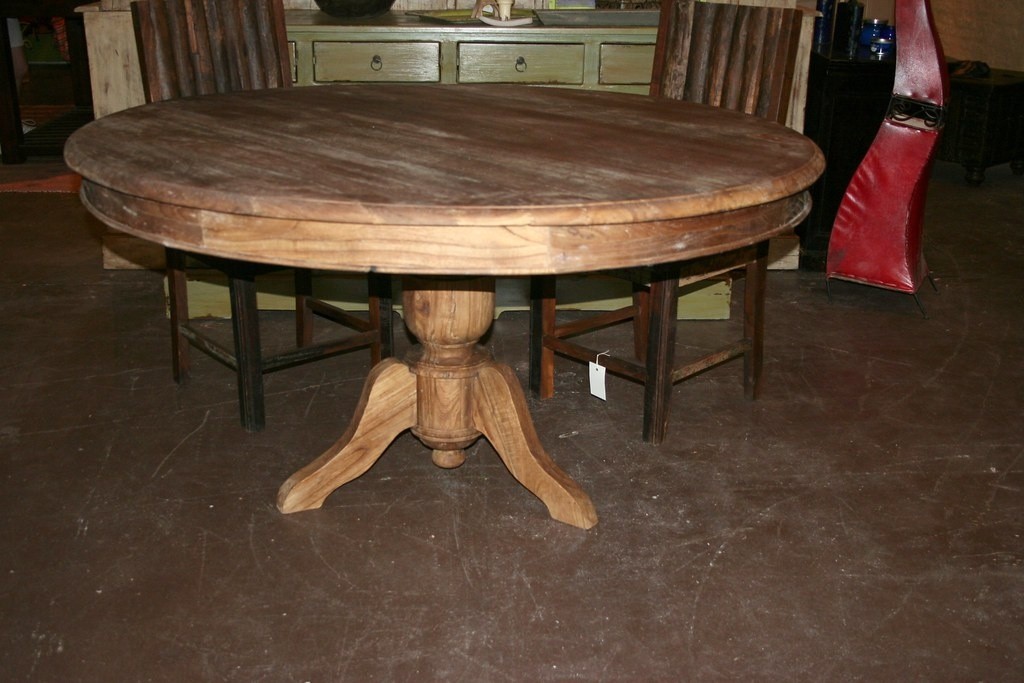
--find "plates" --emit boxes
[404,9,492,25]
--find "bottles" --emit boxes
[860,17,896,60]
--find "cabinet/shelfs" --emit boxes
[796,45,896,257]
[936,66,1024,186]
[158,8,736,320]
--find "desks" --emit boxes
[63,81,826,529]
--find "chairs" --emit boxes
[130,0,393,432]
[530,0,803,448]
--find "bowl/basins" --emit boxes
[314,0,396,17]
